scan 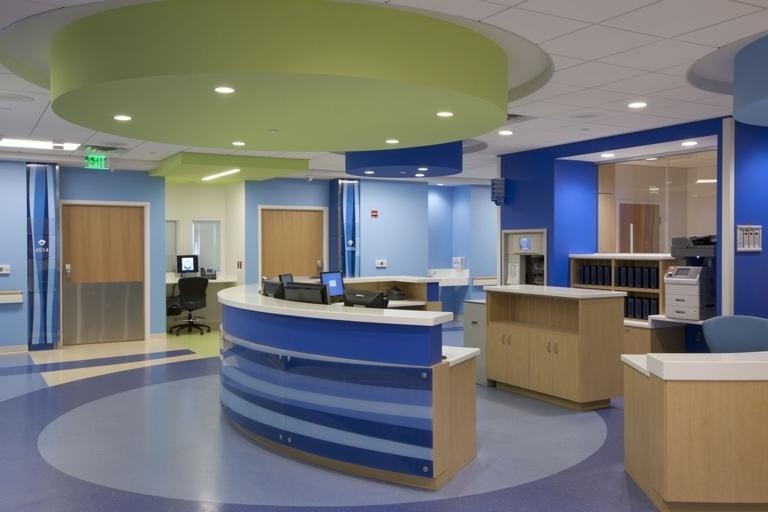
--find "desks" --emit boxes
[427,268,471,287]
[612,342,767,512]
[165,278,238,289]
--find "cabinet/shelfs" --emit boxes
[568,249,691,394]
[483,281,630,413]
[461,297,496,387]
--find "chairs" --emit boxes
[169,275,211,335]
[699,312,767,355]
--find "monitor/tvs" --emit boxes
[284,282,330,304]
[344,289,383,308]
[279,274,293,285]
[176,255,198,272]
[320,271,344,303]
[263,280,285,299]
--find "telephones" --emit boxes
[201,268,216,279]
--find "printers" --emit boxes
[664,234,717,320]
